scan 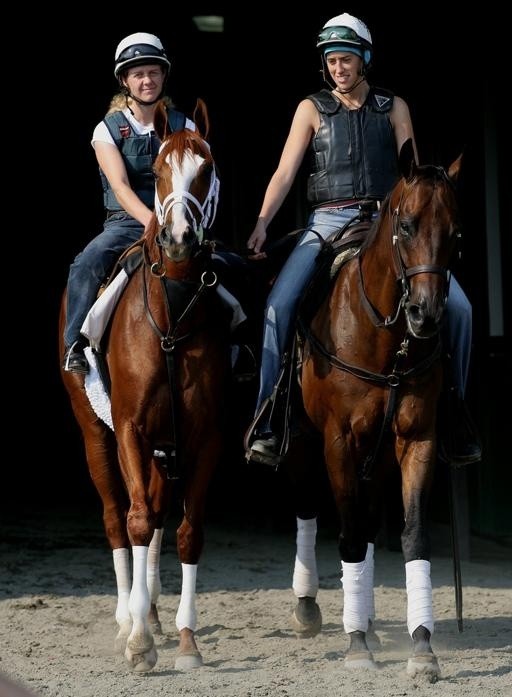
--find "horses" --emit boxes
[58,97,238,674]
[257,138,464,677]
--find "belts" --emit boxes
[338,198,384,211]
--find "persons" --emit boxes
[246,11,482,465]
[59,29,262,391]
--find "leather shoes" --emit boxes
[60,343,91,375]
[251,432,283,460]
[446,435,485,470]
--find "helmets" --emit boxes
[113,32,171,79]
[317,9,373,63]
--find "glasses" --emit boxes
[115,43,164,64]
[316,25,369,44]
